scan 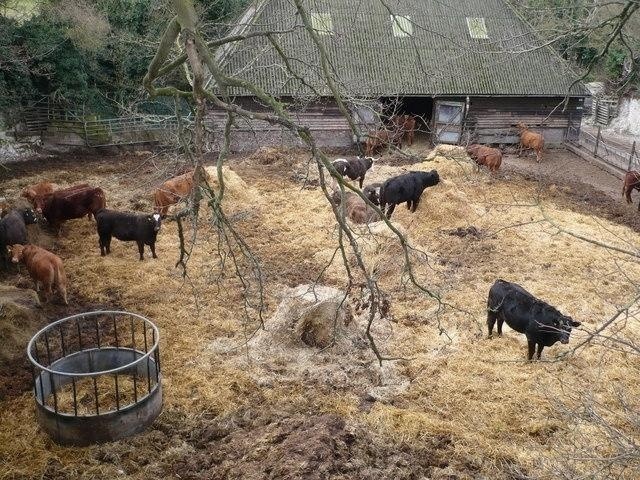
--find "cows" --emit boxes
[388,113,416,147]
[621,170,640,205]
[518,121,546,163]
[379,169,440,221]
[467,142,503,173]
[486,278,581,361]
[366,128,400,156]
[94,208,167,262]
[20,180,107,235]
[154,166,211,218]
[362,182,388,206]
[7,242,69,306]
[0,206,38,271]
[328,154,380,191]
